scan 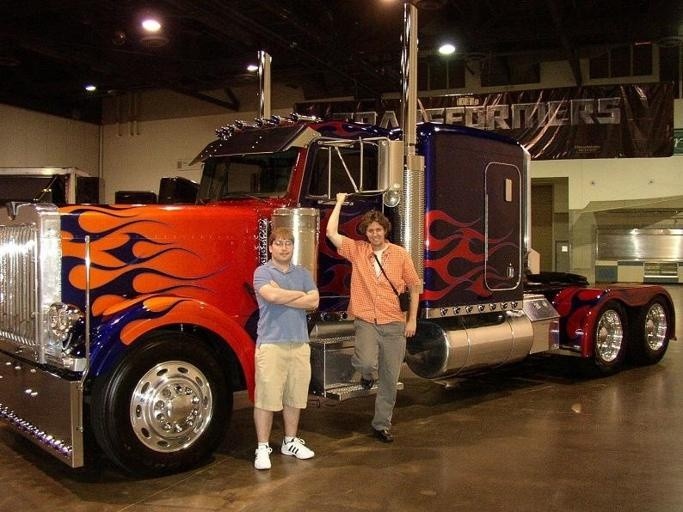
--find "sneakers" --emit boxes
[254,446,273,470]
[280,437,315,459]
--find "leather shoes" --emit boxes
[360,377,374,390]
[374,429,394,443]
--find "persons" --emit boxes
[251,227,322,471]
[325,192,422,444]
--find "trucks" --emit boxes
[0,1,676,478]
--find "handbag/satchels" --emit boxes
[398,292,411,311]
